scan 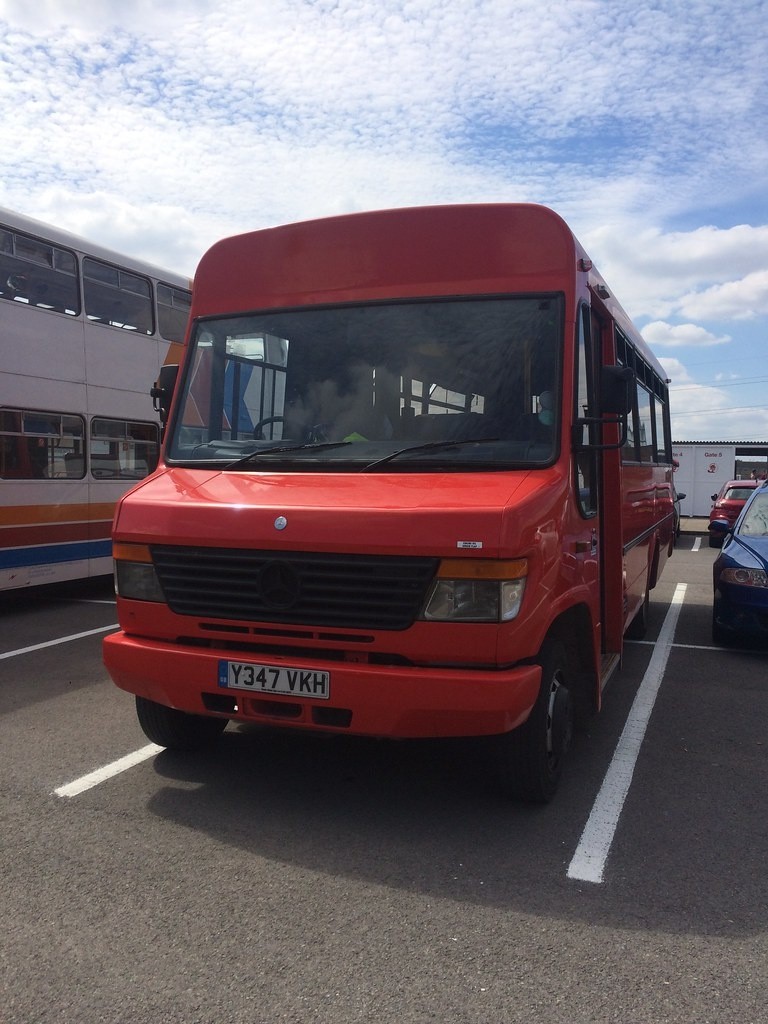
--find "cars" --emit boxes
[712,479,768,641]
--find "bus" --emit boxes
[1,207,287,591]
[106,205,677,806]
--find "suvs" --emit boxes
[708,480,763,547]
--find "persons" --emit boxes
[750,469,756,479]
[760,468,767,479]
